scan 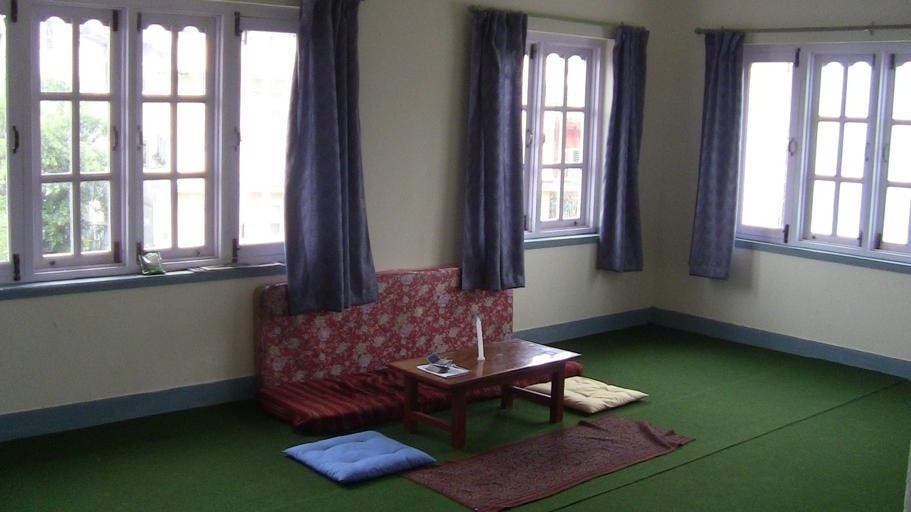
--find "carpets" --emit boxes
[399,410,697,511]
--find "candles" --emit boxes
[476,316,486,360]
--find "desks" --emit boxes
[387,337,583,451]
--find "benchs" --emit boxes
[253,264,586,433]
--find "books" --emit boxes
[416,359,472,380]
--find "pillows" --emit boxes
[281,429,435,484]
[521,375,651,417]
